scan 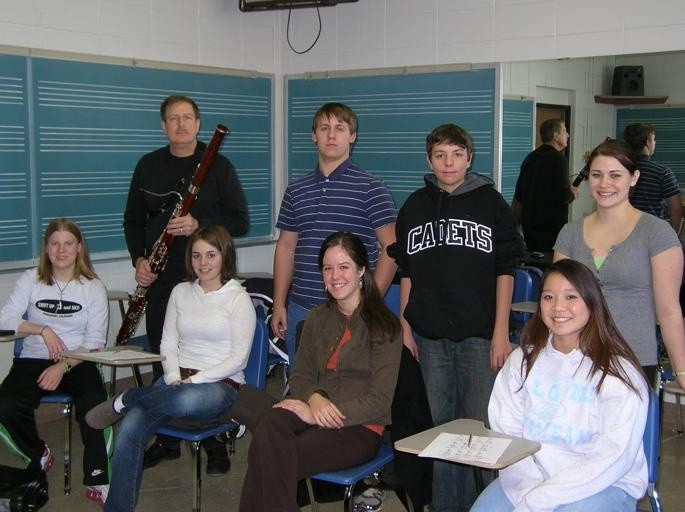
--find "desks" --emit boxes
[57,346,166,400]
[106,290,144,400]
[0,330,31,343]
[394,419,542,495]
[524,251,553,267]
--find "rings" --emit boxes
[180,227,182,233]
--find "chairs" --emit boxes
[305,443,394,512]
[505,266,544,328]
[384,285,418,343]
[155,319,270,512]
[641,383,664,512]
[249,293,291,396]
[14,310,73,496]
[665,379,685,433]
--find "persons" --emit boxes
[123,94,250,475]
[239,230,404,512]
[510,118,581,253]
[551,138,685,395]
[0,218,110,509]
[468,258,649,511]
[624,120,683,368]
[386,122,521,512]
[84,226,257,512]
[269,102,400,371]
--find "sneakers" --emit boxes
[39,445,56,472]
[142,442,181,470]
[84,392,126,430]
[206,444,231,476]
[85,483,111,509]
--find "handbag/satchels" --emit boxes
[9,475,49,512]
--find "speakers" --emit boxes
[612,65,644,95]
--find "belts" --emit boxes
[179,367,240,392]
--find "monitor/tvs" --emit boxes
[239,0,362,12]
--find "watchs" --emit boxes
[63,360,73,374]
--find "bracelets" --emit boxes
[671,370,685,377]
[38,326,48,336]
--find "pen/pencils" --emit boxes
[468,432,472,448]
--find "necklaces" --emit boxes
[52,274,74,297]
